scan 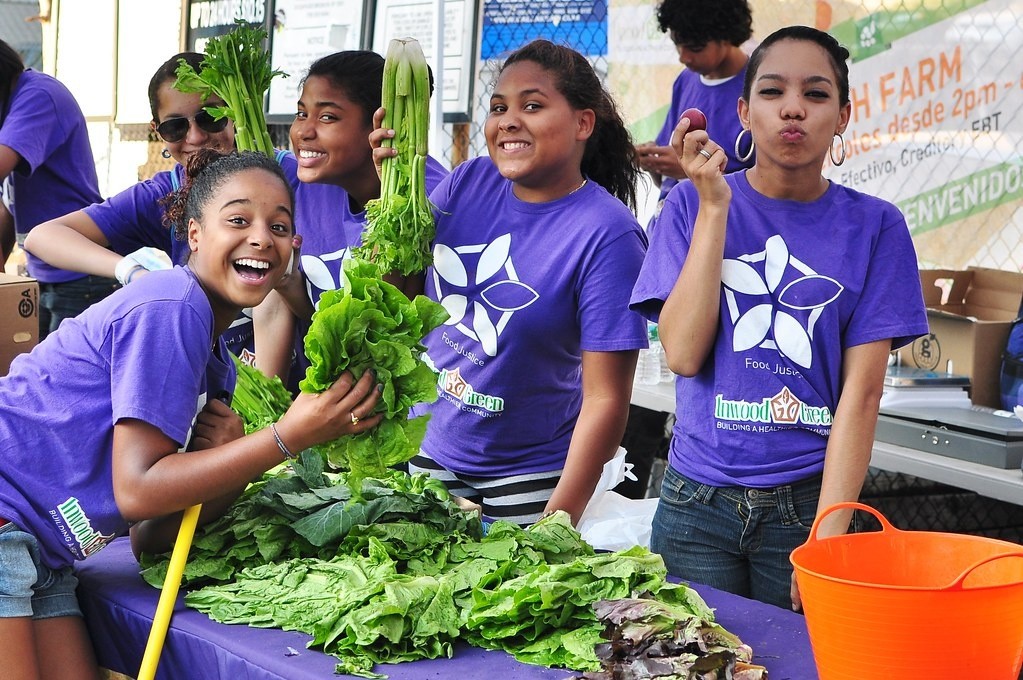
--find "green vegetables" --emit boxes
[133,256,772,680]
[172,18,290,158]
[350,36,453,276]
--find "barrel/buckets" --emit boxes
[788,502,1023,680]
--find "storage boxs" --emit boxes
[0,273,41,379]
[889,266,1023,408]
[874,405,1023,470]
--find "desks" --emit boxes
[72,534,823,680]
[611,374,1023,507]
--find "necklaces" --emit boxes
[570,180,587,195]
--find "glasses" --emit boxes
[156,106,229,143]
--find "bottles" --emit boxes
[634,315,674,388]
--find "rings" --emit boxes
[351,411,359,425]
[700,149,711,159]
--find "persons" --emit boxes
[632,0,757,244]
[0,146,390,680]
[369,39,651,530]
[0,38,116,343]
[24,50,450,399]
[626,26,931,615]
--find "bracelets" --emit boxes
[127,267,144,283]
[115,256,139,286]
[270,423,296,459]
